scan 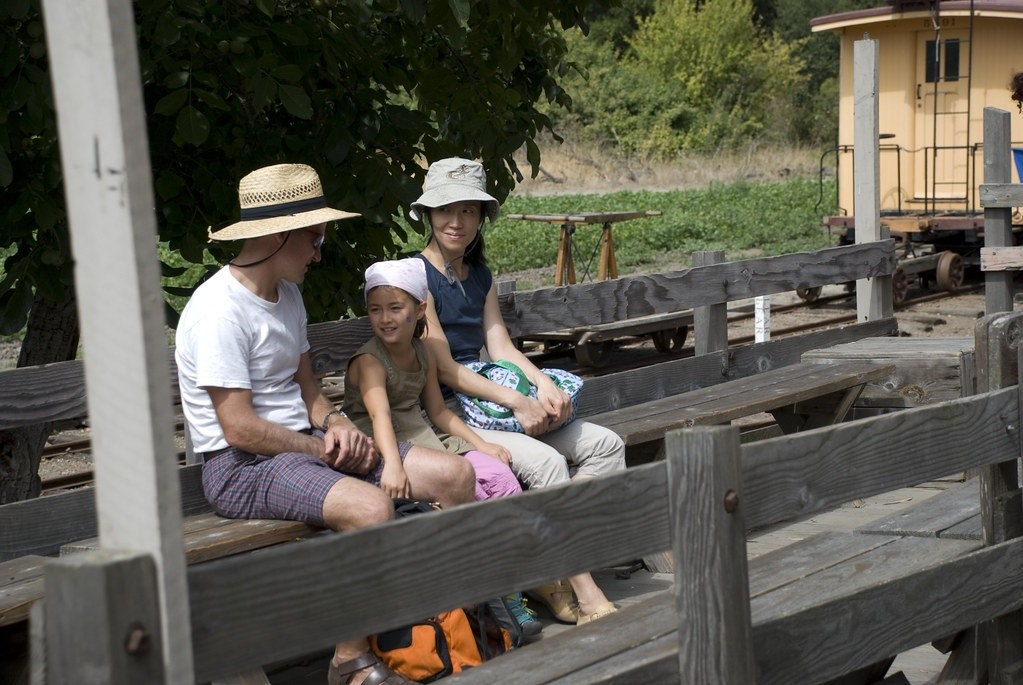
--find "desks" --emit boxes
[508,208,662,284]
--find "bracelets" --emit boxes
[322,410,348,434]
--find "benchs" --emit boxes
[0,237,899,630]
[33,310,1022,684]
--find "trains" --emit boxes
[809,1,1023,310]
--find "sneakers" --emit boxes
[507,590,543,637]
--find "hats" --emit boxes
[364,258,428,306]
[208,163,362,241]
[411,158,500,223]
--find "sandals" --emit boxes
[326,651,422,684]
[526,577,618,627]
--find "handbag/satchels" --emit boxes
[456,360,582,435]
[371,597,521,683]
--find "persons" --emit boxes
[408,153,626,628]
[341,255,546,639]
[171,163,478,684]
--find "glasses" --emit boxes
[302,228,324,249]
